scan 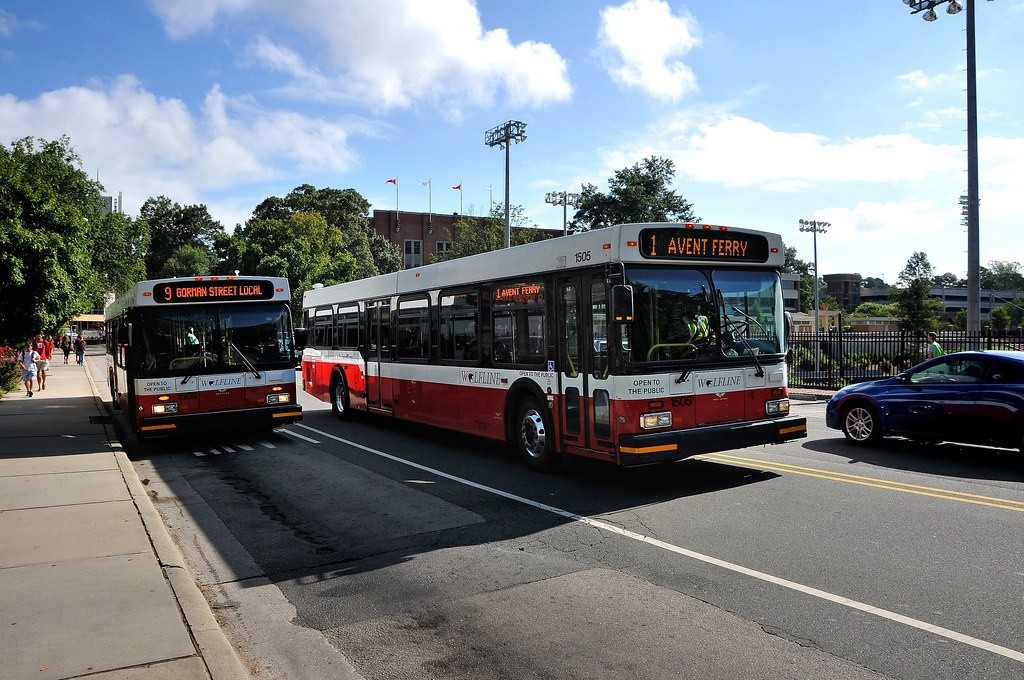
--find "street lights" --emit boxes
[798,217,831,372]
[543,190,588,242]
[483,119,530,250]
[899,0,989,349]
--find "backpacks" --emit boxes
[76,340,85,353]
[932,342,944,357]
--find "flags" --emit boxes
[484,188,491,191]
[386,179,396,184]
[452,184,461,190]
[422,181,429,186]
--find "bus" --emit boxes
[292,221,808,477]
[103,274,309,445]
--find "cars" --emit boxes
[824,349,1024,452]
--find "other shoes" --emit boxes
[26,390,30,396]
[29,391,33,397]
[42,383,45,390]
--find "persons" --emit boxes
[48,336,55,362]
[928,332,943,360]
[671,299,716,357]
[187,326,199,344]
[62,336,71,364]
[58,334,63,349]
[32,331,51,391]
[73,335,85,366]
[18,341,40,397]
